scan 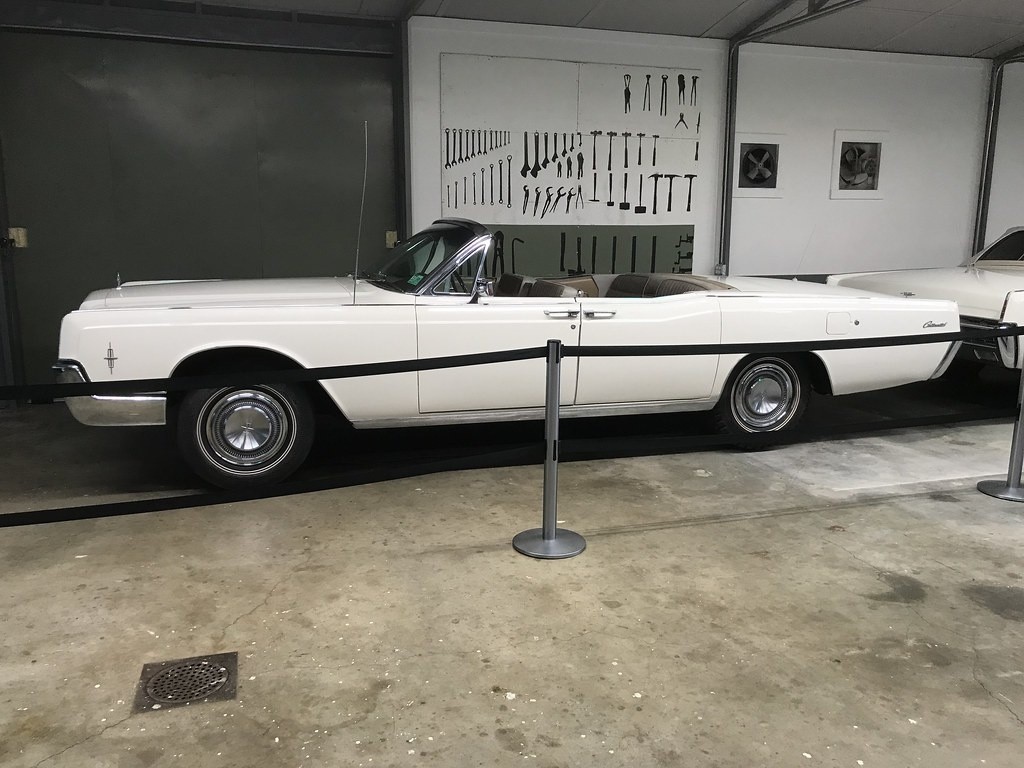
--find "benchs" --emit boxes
[606,272,722,297]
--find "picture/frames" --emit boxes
[732,132,787,198]
[830,129,887,199]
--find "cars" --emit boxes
[51,217,963,494]
[824,226,1024,386]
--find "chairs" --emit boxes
[499,273,580,297]
